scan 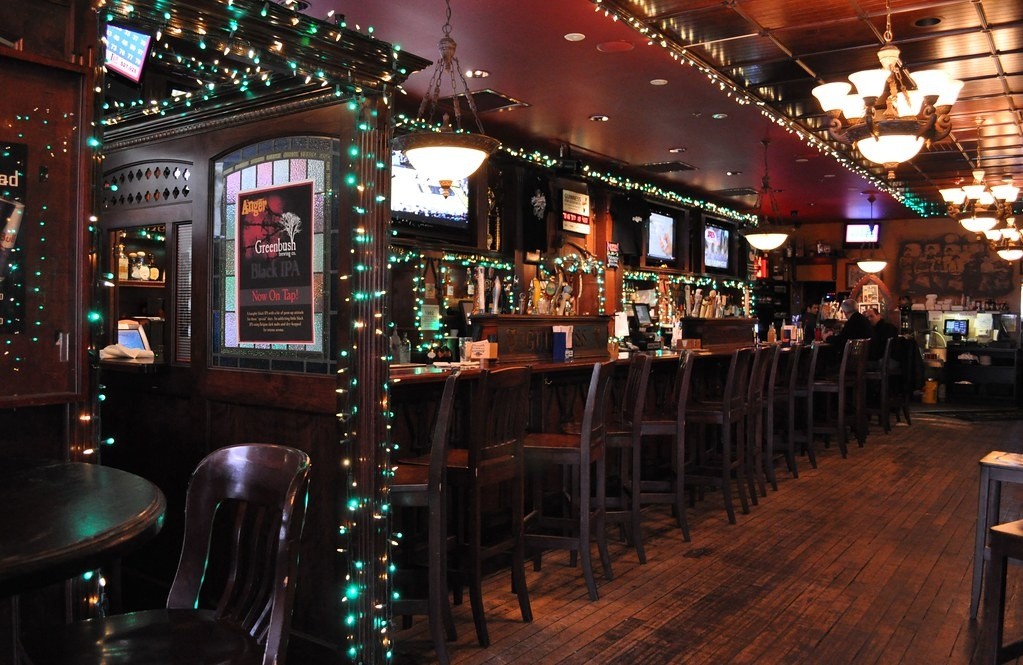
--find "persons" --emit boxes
[823,299,876,362]
[865,308,898,357]
[803,301,819,343]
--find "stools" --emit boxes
[388,335,918,665]
[971,449,1023,665]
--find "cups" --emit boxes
[975,300,981,311]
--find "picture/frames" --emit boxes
[845,263,884,290]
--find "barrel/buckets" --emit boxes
[921,379,937,403]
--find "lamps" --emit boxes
[291,0,345,42]
[737,139,796,252]
[389,0,499,200]
[810,0,964,180]
[938,116,1023,265]
[857,198,890,273]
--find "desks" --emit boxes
[0,455,166,665]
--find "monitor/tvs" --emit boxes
[842,222,882,249]
[644,208,679,267]
[704,221,733,274]
[632,303,652,326]
[943,319,969,341]
[389,147,478,246]
[118,323,150,352]
[458,300,473,324]
[103,25,154,89]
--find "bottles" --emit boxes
[985,298,1009,311]
[389,324,451,365]
[119,244,160,282]
[767,321,803,347]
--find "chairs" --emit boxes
[18,442,311,665]
